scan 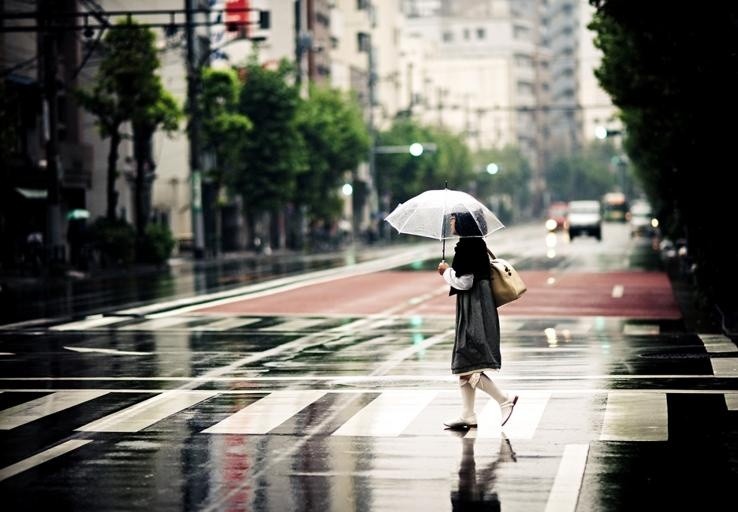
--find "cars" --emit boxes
[546,192,629,242]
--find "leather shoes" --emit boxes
[443,413,479,428]
[499,393,519,427]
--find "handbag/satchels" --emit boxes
[486,248,528,310]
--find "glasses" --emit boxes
[448,216,457,220]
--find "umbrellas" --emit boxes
[382,180,507,266]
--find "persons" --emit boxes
[444,427,519,512]
[437,206,518,430]
